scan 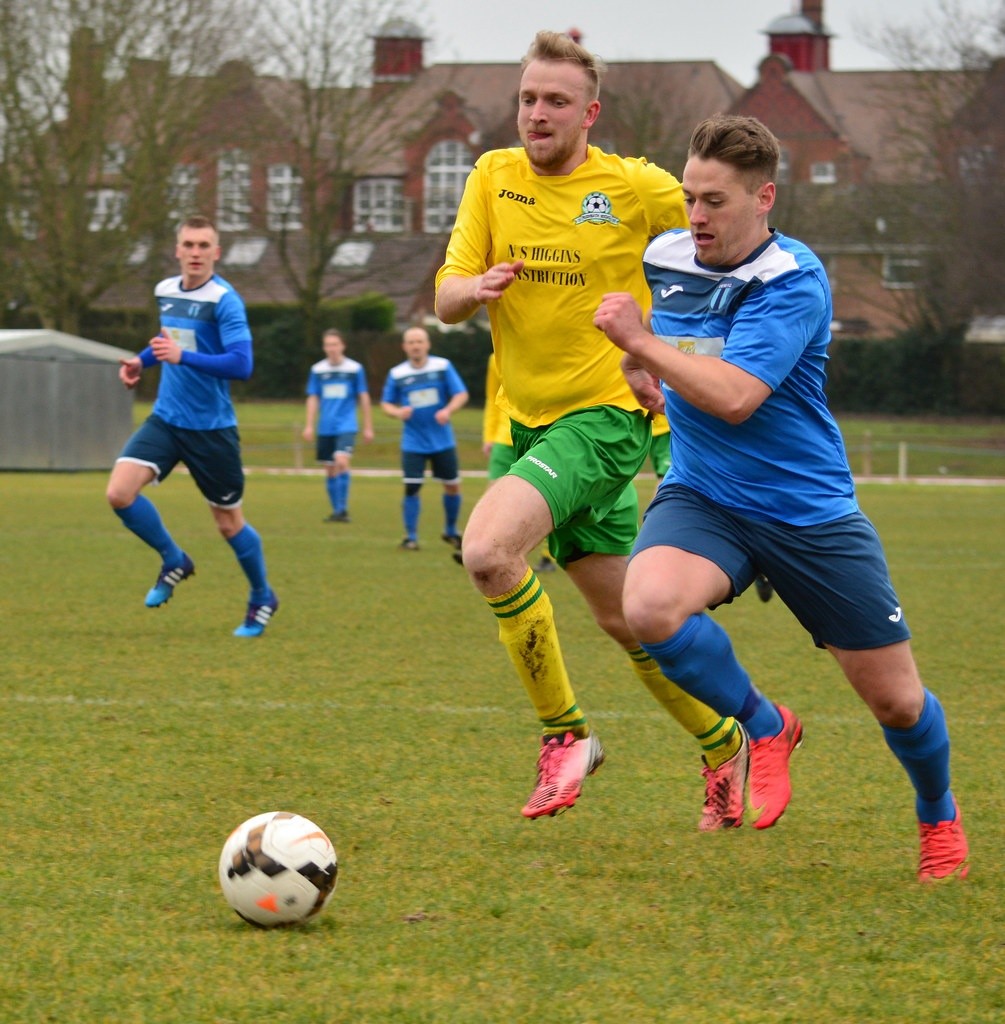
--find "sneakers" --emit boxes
[233,590,279,636]
[749,701,805,830]
[917,793,969,882]
[145,553,195,608]
[520,724,606,820]
[697,719,751,829]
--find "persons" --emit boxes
[303,330,373,523]
[591,113,970,891]
[107,217,279,638]
[435,30,747,832]
[648,407,674,479]
[381,328,472,549]
[452,350,554,567]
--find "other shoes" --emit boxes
[400,538,418,552]
[442,533,462,550]
[327,511,349,520]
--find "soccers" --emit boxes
[217,810,340,930]
